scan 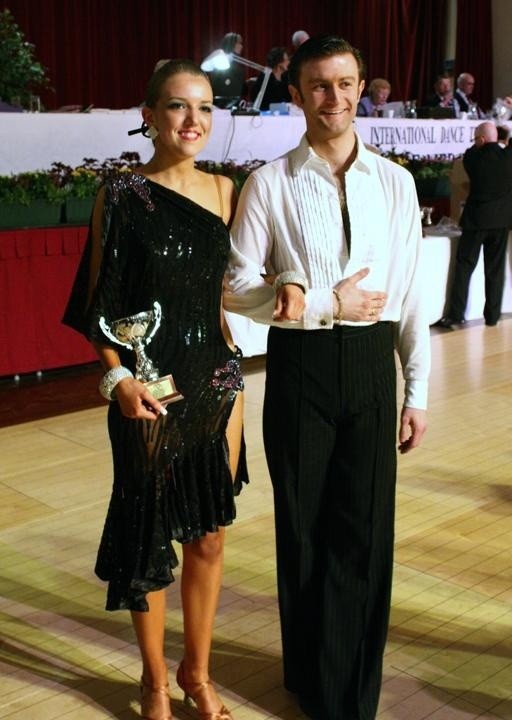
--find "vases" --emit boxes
[66,196,95,224]
[0,199,65,228]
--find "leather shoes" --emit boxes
[430,316,466,327]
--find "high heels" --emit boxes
[139,676,173,719]
[176,661,233,720]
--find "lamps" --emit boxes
[202,50,273,109]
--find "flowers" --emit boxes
[382,149,451,178]
[0,160,66,202]
[193,159,267,191]
[65,158,133,197]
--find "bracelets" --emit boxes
[98,362,133,402]
[273,271,307,292]
[332,289,344,320]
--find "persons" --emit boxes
[429,97,512,327]
[210,30,490,118]
[219,38,432,719]
[63,62,306,720]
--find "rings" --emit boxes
[371,308,377,316]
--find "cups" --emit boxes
[11,97,21,106]
[388,109,394,119]
[459,111,466,121]
[32,95,40,113]
